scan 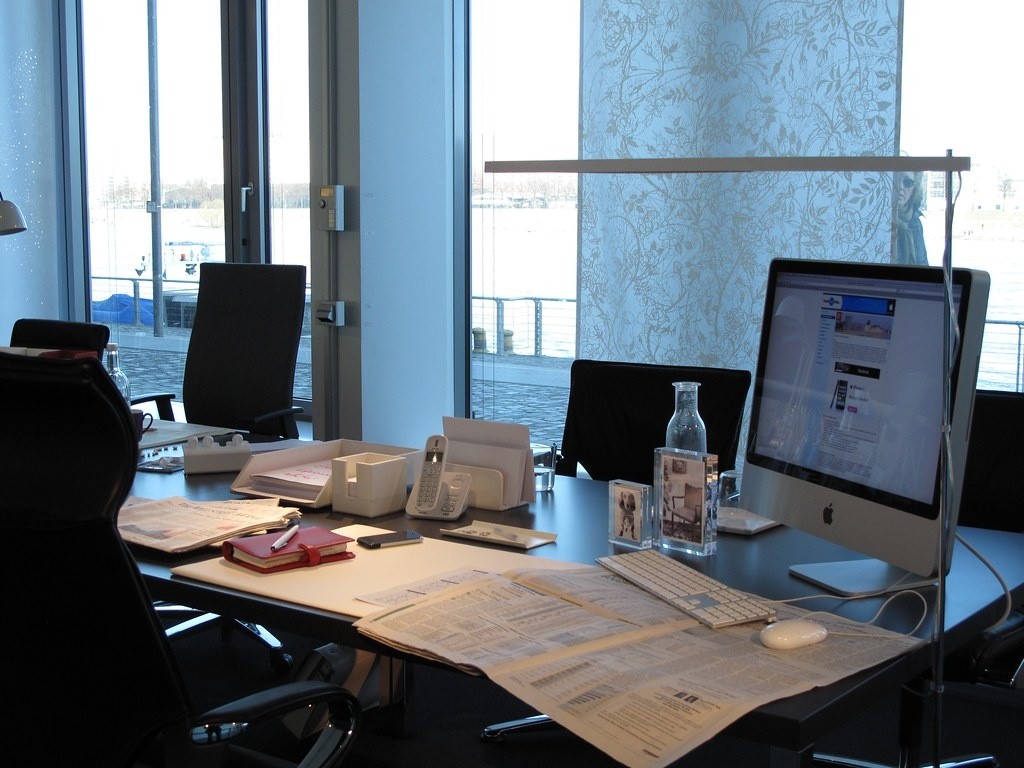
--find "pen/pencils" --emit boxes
[271,524,300,551]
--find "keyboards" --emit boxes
[595,548,778,628]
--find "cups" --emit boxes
[529,442,557,492]
[130,409,154,443]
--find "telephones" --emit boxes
[405,434,473,520]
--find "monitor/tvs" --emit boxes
[742,258,990,597]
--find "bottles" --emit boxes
[103,343,131,409]
[666,380,708,453]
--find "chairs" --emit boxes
[10,318,111,363]
[812,392,1024,768]
[482,362,752,743]
[128,264,307,677]
[672,482,702,542]
[0,351,360,768]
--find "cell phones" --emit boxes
[357,529,423,549]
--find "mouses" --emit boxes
[761,619,827,649]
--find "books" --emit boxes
[221,526,356,574]
[250,459,332,501]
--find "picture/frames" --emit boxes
[672,459,686,473]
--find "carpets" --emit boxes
[663,520,701,542]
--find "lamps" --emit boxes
[0,193,26,236]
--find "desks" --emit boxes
[130,433,1024,768]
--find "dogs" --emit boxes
[618,490,638,540]
